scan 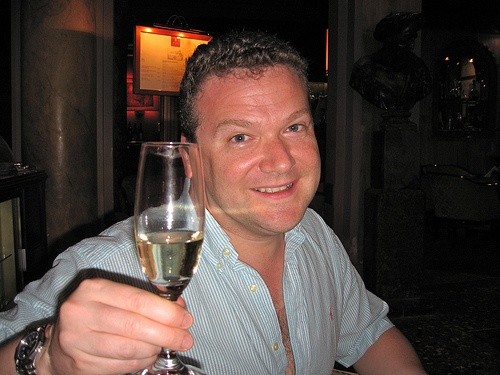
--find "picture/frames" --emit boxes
[126,73,160,112]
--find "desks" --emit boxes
[0,167,51,287]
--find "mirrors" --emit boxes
[432,37,496,139]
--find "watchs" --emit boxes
[13,319,54,375]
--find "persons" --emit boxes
[0,28,428,375]
[348,10,434,128]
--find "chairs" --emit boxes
[420,162,500,251]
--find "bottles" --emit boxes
[127,121,144,146]
[307,88,327,124]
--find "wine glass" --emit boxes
[133,142,206,375]
